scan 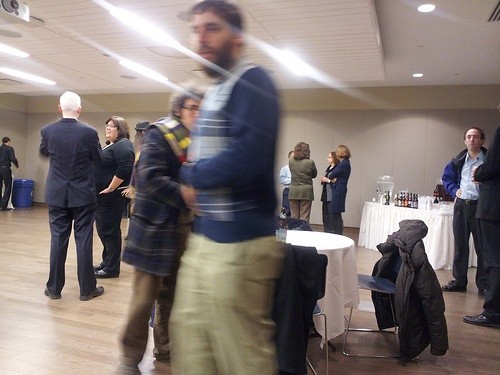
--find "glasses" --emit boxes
[181,104,199,115]
[105,124,117,128]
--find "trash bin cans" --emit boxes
[12,178,35,207]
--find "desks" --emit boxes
[358,196,478,271]
[276,230,359,350]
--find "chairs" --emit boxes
[288,275,329,375]
[341,219,426,361]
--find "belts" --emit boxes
[0,166,10,168]
[457,197,478,206]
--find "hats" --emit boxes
[134,121,150,131]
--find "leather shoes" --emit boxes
[477,289,490,297]
[463,312,500,329]
[44,287,61,299]
[93,269,119,278]
[92,265,102,271]
[79,286,104,301]
[441,279,467,292]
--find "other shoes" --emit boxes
[0,207,10,211]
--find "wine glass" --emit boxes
[437,197,443,207]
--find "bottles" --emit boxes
[278,207,288,242]
[395,192,418,209]
[382,191,390,205]
[433,190,439,203]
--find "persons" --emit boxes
[169,1,279,375]
[0,137,19,211]
[280,151,295,218]
[135,121,150,154]
[442,127,488,296]
[288,142,317,224]
[92,116,135,278]
[320,152,340,233]
[39,91,103,301]
[463,123,500,328]
[329,145,351,235]
[114,87,201,375]
